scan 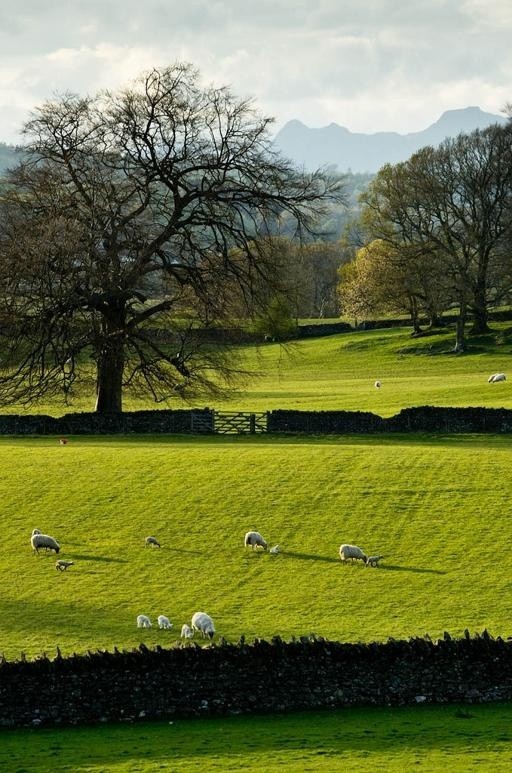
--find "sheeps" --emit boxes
[138,612,155,630]
[33,529,41,535]
[493,374,506,383]
[31,533,62,556]
[488,373,498,383]
[270,544,280,554]
[55,560,74,572]
[339,544,368,564]
[146,537,162,550]
[365,554,385,569]
[158,614,175,632]
[179,624,198,643]
[244,531,268,555]
[192,611,218,641]
